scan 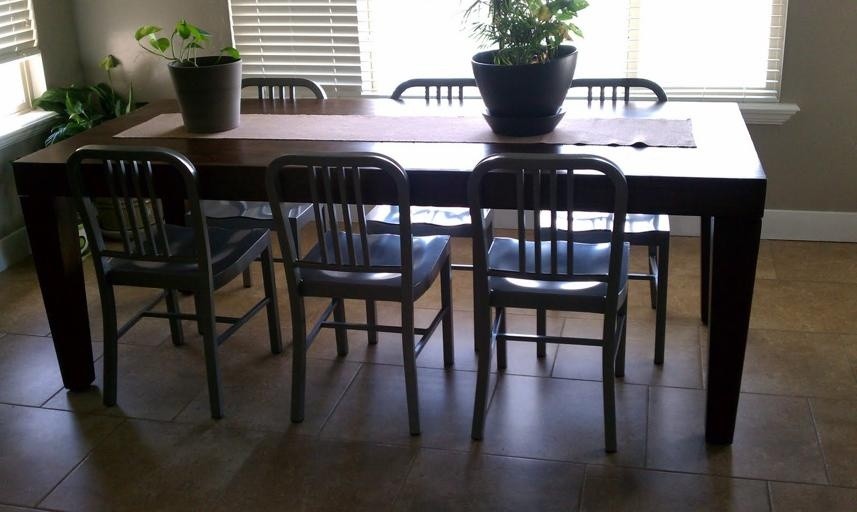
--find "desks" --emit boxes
[10,97,768,446]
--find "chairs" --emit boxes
[64,142,283,423]
[468,152,633,453]
[533,77,674,370]
[263,152,458,440]
[201,77,333,292]
[356,77,492,272]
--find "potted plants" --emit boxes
[132,18,243,134]
[31,53,163,241]
[458,0,589,138]
